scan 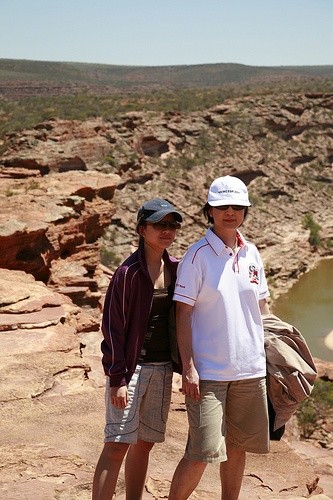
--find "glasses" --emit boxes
[214,206,245,211]
[144,222,182,231]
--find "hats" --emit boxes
[136,198,183,222]
[207,176,252,206]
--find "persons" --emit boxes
[168,175,271,500]
[92,199,181,500]
